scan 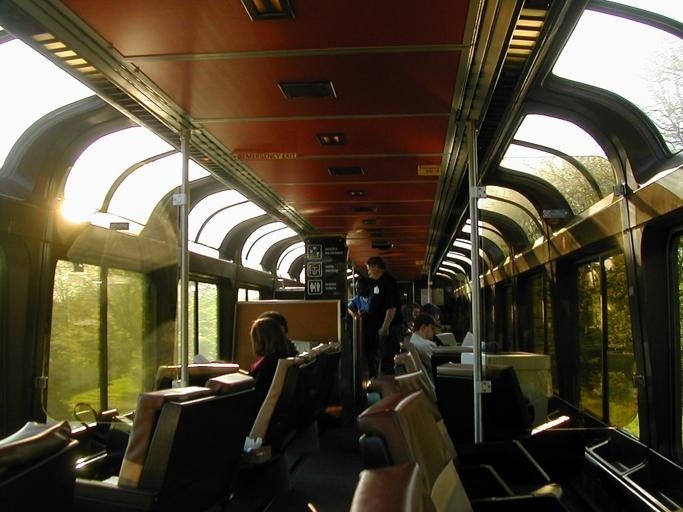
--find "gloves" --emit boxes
[378,313,396,337]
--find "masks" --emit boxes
[367,267,379,280]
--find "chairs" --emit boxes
[0,328,683,512]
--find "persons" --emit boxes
[365,255,402,399]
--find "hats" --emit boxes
[414,313,442,330]
